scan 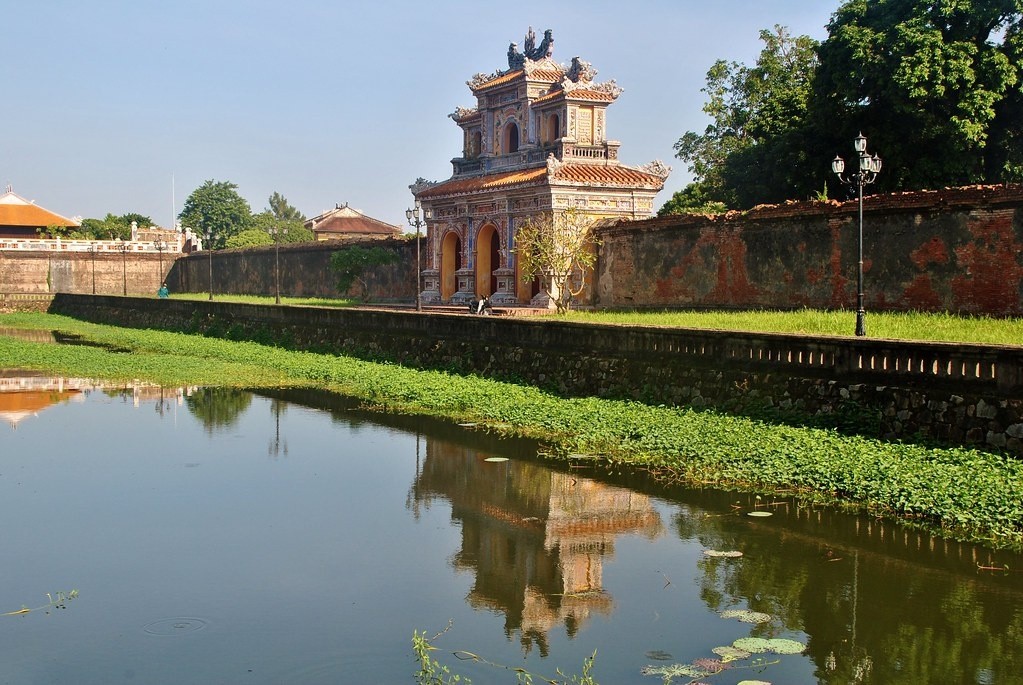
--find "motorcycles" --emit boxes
[470,294,493,316]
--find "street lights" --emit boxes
[87,245,101,294]
[153,234,167,287]
[267,224,289,303]
[118,241,131,295]
[201,227,218,299]
[832,131,882,336]
[406,197,433,311]
